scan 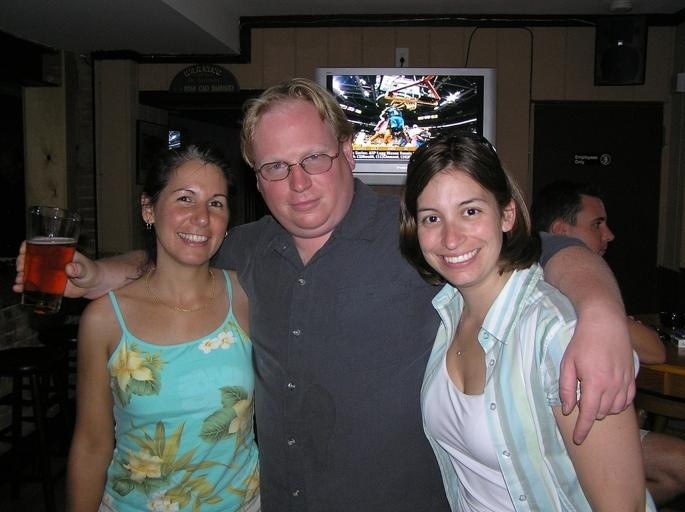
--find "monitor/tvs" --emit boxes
[312,64,499,188]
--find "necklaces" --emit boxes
[455,314,484,356]
[145,267,217,313]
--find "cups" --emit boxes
[21,205,84,315]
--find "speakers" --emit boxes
[593,16,650,87]
[18,46,61,87]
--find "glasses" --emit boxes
[416,132,501,166]
[252,135,342,182]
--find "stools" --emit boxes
[30,301,91,415]
[0,347,71,512]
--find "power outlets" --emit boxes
[395,48,410,67]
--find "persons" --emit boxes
[11,75,638,511]
[398,131,660,511]
[355,102,431,146]
[65,139,261,511]
[530,182,685,511]
